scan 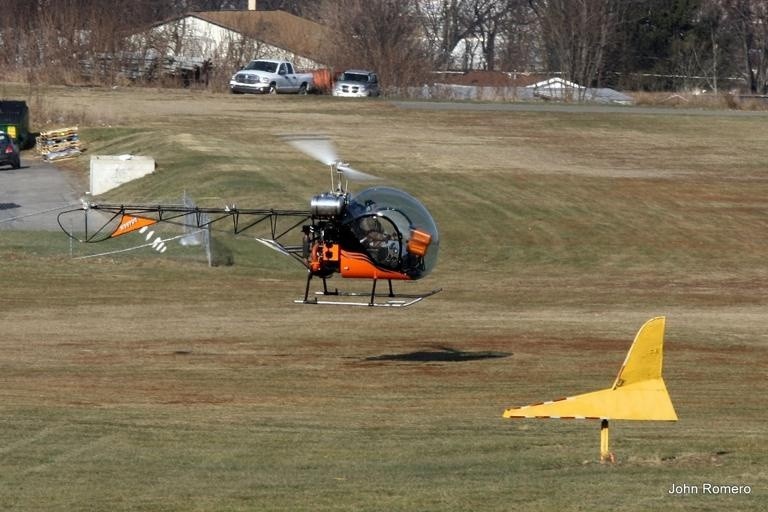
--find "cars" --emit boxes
[1,99,35,169]
[332,69,380,97]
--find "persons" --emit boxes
[358,199,409,259]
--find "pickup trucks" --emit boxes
[229,58,313,95]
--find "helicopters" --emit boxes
[56,140,443,307]
